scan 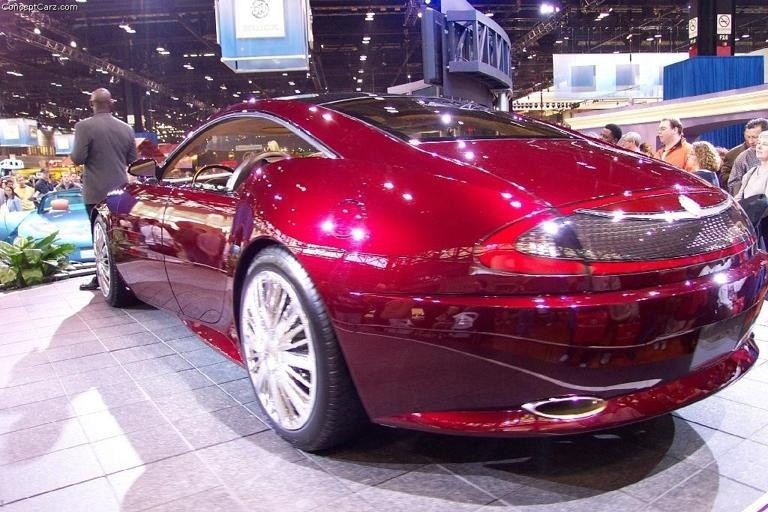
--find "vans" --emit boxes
[0,159,24,170]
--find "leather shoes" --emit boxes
[79,280,99,290]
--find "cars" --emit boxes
[87,91,768,456]
[0,190,97,262]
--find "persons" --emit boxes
[69,87,138,291]
[595,115,767,255]
[0,166,85,212]
